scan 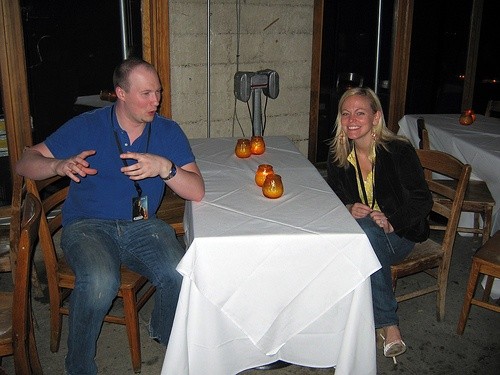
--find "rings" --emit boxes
[381,221,383,224]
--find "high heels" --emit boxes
[384,338,406,364]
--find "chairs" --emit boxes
[417,118,495,243]
[382,145,473,323]
[0,174,152,375]
[456,229,500,332]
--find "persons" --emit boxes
[327,87,433,365]
[16,59,205,375]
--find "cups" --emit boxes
[255,165,274,187]
[461,109,476,126]
[235,139,251,158]
[263,173,284,199]
[250,136,265,155]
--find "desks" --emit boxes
[159,136,381,374]
[399,111,500,241]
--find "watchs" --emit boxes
[160,160,177,180]
